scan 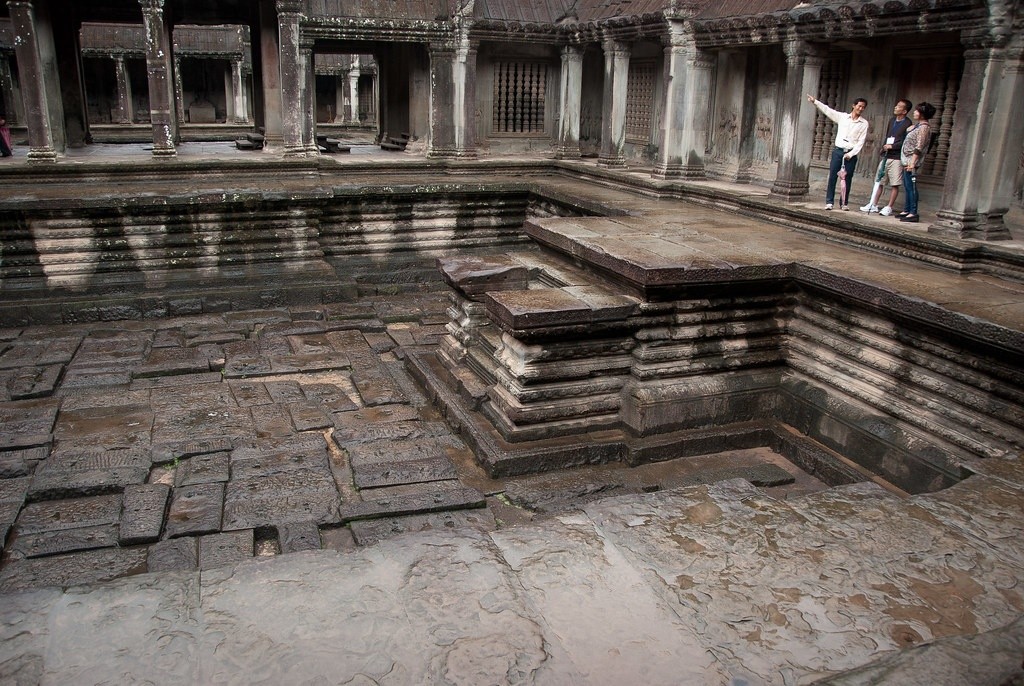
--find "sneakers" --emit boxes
[878,205,893,216]
[859,203,879,213]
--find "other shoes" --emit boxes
[825,202,834,210]
[899,215,920,222]
[895,210,909,218]
[840,205,850,211]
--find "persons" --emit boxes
[860,100,913,216]
[807,94,869,211]
[895,102,936,223]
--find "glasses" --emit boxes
[921,101,926,112]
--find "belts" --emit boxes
[835,145,852,153]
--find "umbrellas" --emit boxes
[868,148,888,215]
[837,156,847,211]
